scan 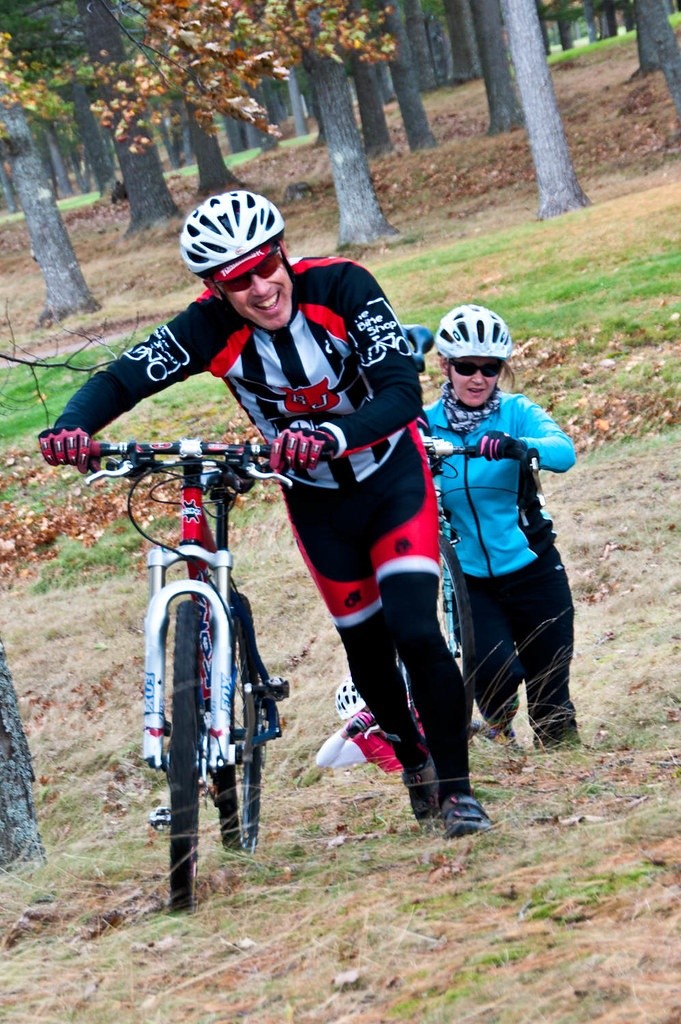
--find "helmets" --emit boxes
[179,190,286,280]
[434,304,513,361]
[334,677,367,721]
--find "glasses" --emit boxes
[437,351,505,377]
[213,246,284,293]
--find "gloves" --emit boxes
[345,713,375,739]
[38,427,102,475]
[476,430,527,462]
[269,427,338,476]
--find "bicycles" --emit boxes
[347,651,502,739]
[89,440,334,916]
[403,322,547,740]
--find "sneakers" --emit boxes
[401,755,442,834]
[503,724,519,755]
[440,795,493,840]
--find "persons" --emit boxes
[111,181,127,203]
[35,189,491,839]
[417,304,579,751]
[315,673,426,775]
